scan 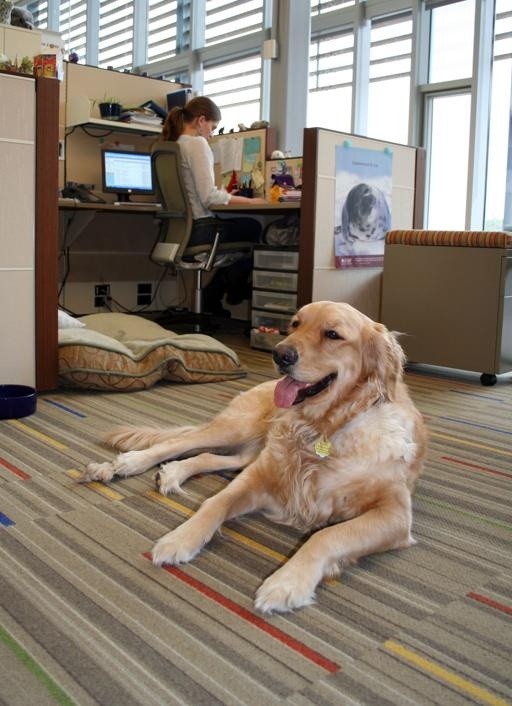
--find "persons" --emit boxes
[161,96,261,317]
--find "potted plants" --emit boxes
[92,91,124,120]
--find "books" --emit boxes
[123,100,167,128]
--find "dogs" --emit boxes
[78,301,429,614]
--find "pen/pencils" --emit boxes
[243,180,251,189]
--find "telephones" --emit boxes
[62,186,106,203]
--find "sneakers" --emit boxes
[195,296,235,321]
[222,280,252,307]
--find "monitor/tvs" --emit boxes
[101,149,155,202]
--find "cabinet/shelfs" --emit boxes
[376,228,512,386]
[246,244,300,353]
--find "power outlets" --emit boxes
[94,284,110,295]
[94,296,105,306]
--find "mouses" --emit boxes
[114,202,120,207]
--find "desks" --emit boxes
[59,197,305,219]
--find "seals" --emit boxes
[341,183,392,246]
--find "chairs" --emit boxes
[146,138,258,336]
